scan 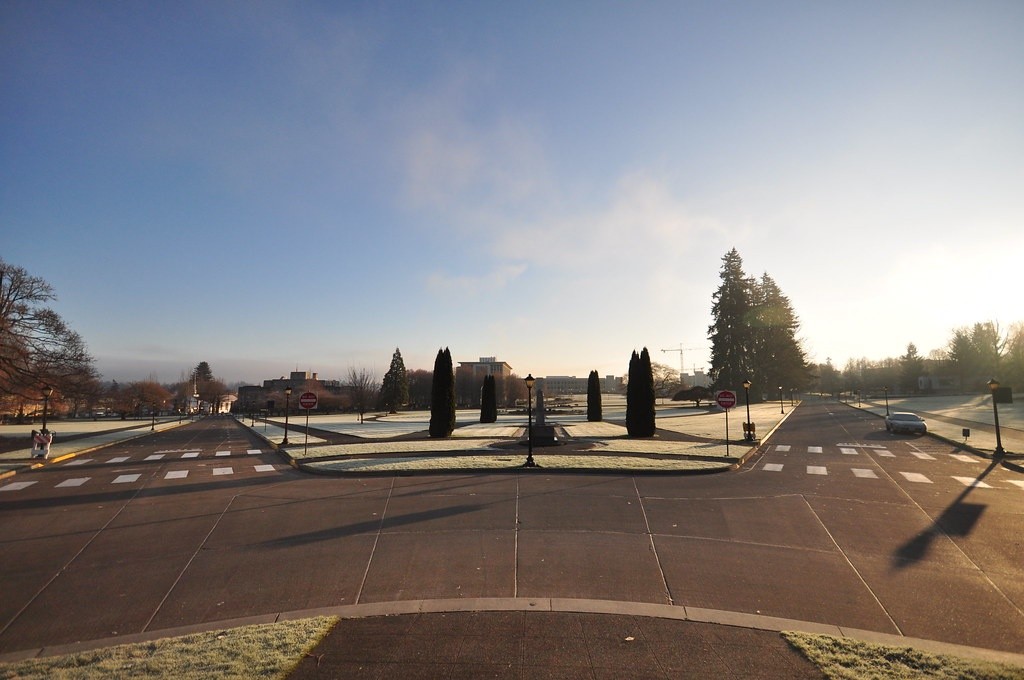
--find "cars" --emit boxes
[885,410,927,435]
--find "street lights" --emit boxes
[986,379,1008,456]
[283,383,292,445]
[152,401,156,430]
[777,383,785,414]
[790,386,795,406]
[523,373,538,466]
[883,385,892,416]
[38,384,56,458]
[251,398,257,428]
[856,388,861,407]
[741,379,754,441]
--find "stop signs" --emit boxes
[715,390,738,408]
[299,390,319,410]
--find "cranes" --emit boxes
[660,343,705,374]
